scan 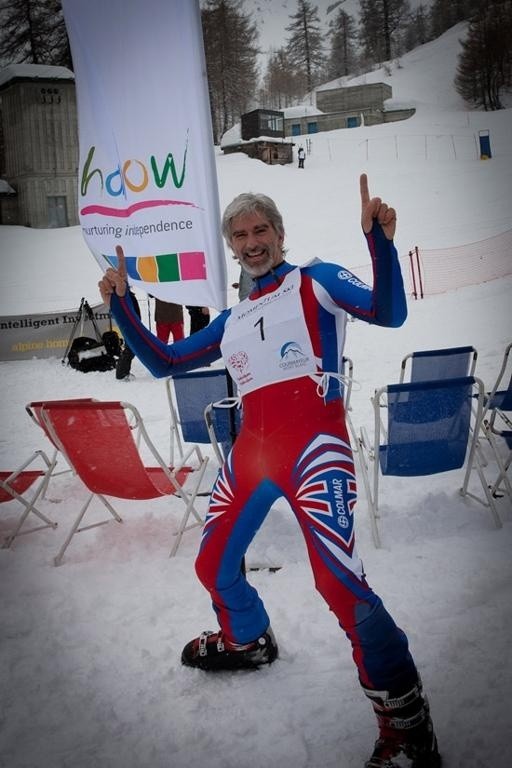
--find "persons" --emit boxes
[298,148,301,167]
[185,306,210,367]
[300,148,305,168]
[98,174,442,768]
[149,294,184,345]
[116,283,141,381]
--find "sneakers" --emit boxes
[181,624,278,671]
[365,731,442,767]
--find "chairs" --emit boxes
[41,400,210,562]
[2,450,63,546]
[359,376,495,548]
[27,395,99,501]
[167,364,239,467]
[338,361,378,548]
[169,402,245,557]
[376,345,511,500]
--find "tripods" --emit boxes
[61,296,103,363]
